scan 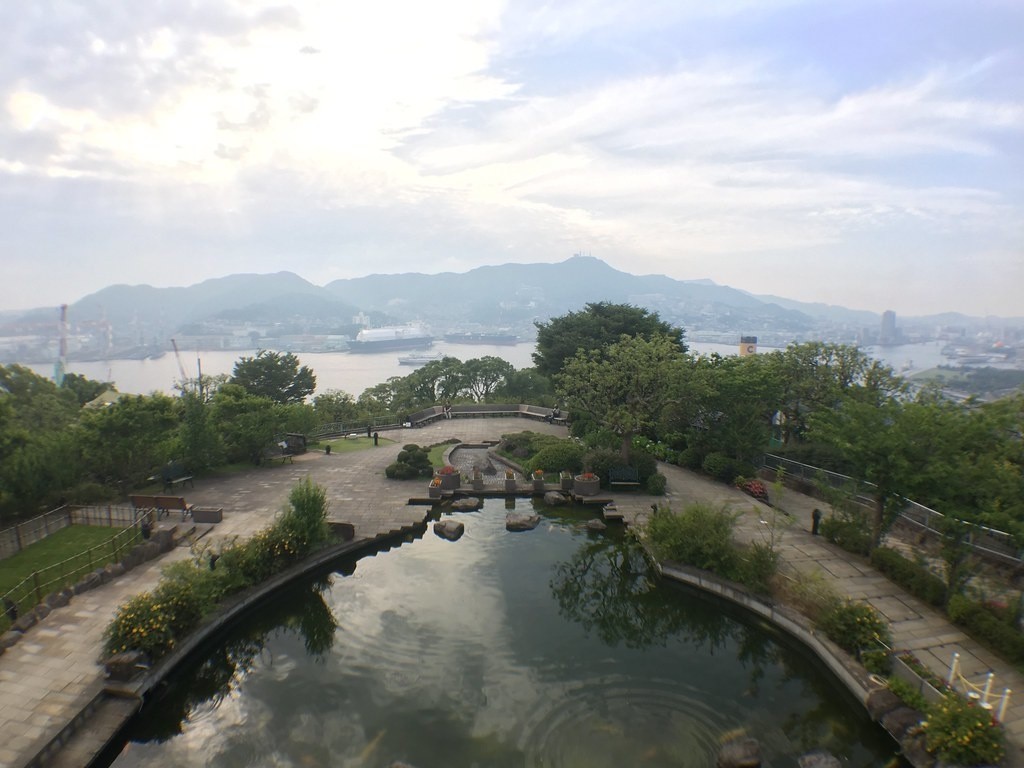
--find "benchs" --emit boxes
[160,464,194,495]
[608,467,641,493]
[407,404,569,429]
[263,446,293,467]
[129,494,194,523]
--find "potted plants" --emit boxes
[560,469,572,489]
[473,468,484,490]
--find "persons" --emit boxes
[406,414,414,428]
[545,403,560,424]
[443,400,452,419]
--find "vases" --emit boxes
[921,680,960,711]
[574,475,601,495]
[435,470,460,490]
[191,506,222,524]
[429,480,443,498]
[532,474,544,490]
[505,472,516,489]
[892,655,929,695]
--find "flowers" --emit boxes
[928,676,969,705]
[577,473,597,481]
[900,649,933,679]
[506,469,514,479]
[439,466,458,476]
[433,476,441,486]
[534,470,543,479]
